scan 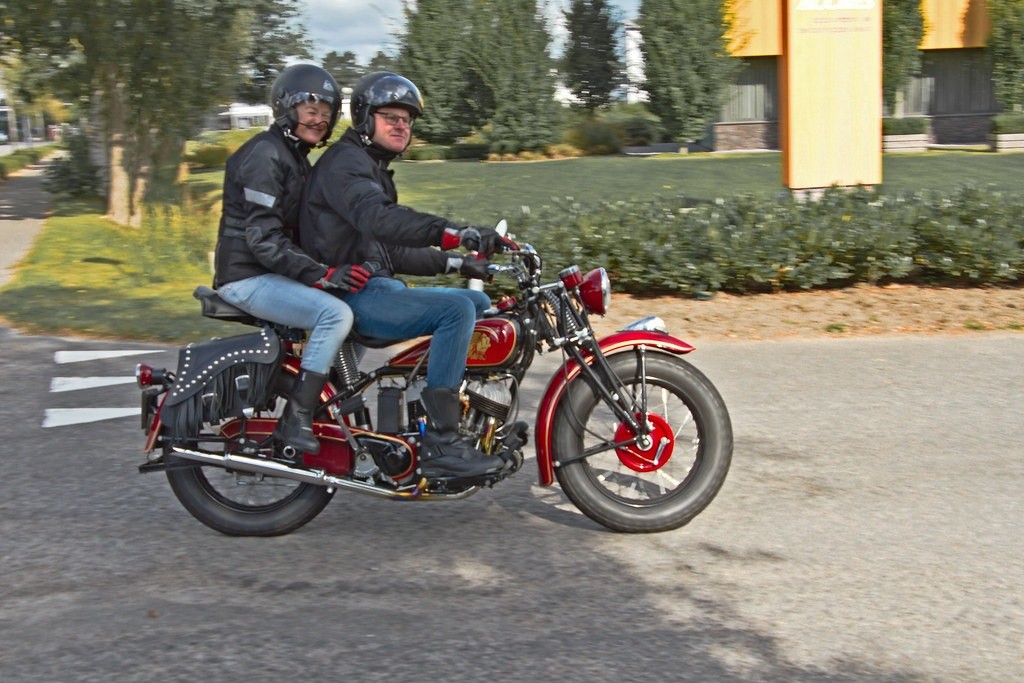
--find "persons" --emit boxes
[299,71,520,478]
[212,64,371,454]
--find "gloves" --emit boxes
[311,264,371,293]
[444,248,493,284]
[441,221,520,260]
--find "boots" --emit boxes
[421,386,504,478]
[272,367,329,455]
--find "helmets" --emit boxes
[350,71,423,142]
[271,64,342,148]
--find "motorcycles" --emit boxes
[133,204,735,539]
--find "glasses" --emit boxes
[373,111,415,128]
